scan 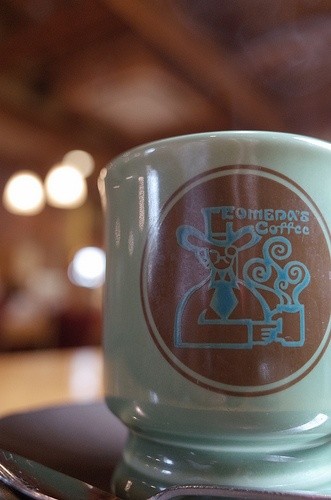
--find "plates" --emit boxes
[0,399,130,500]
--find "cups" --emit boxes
[97,129,330,492]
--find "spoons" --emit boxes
[0,450,329,500]
